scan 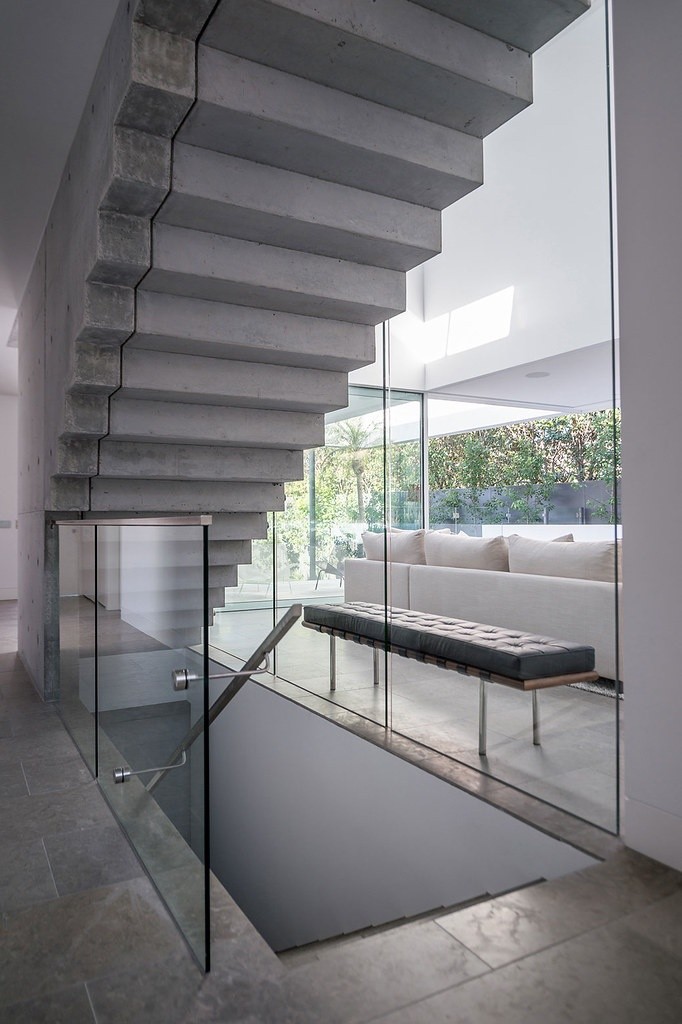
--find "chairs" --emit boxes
[313,559,345,591]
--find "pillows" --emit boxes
[361,529,425,565]
[552,534,575,542]
[387,525,451,534]
[423,530,509,573]
[506,533,622,584]
[458,530,509,544]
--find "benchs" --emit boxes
[302,600,598,757]
[236,567,293,598]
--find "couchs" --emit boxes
[346,558,623,682]
